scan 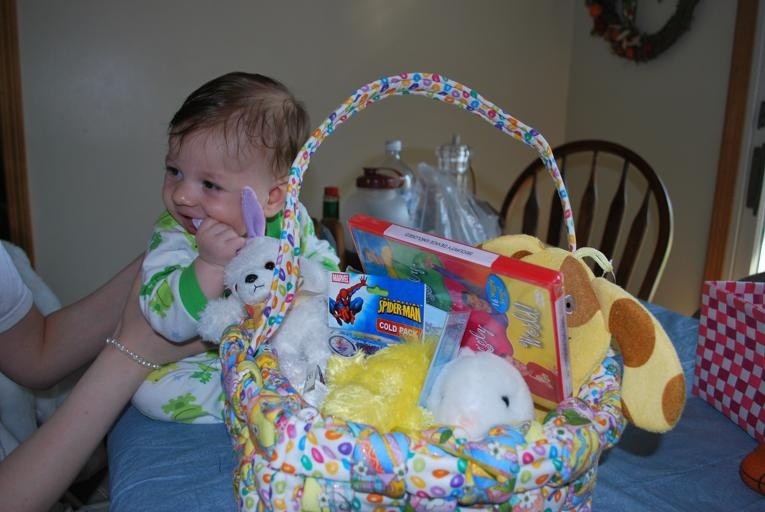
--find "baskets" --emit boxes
[218,73,628,511]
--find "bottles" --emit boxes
[316,185,343,264]
[342,133,500,270]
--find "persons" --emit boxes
[125,68,360,426]
[0,208,219,511]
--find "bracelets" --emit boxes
[102,335,165,370]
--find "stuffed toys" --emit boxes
[190,187,337,404]
[318,332,438,436]
[471,233,685,455]
[419,342,536,436]
